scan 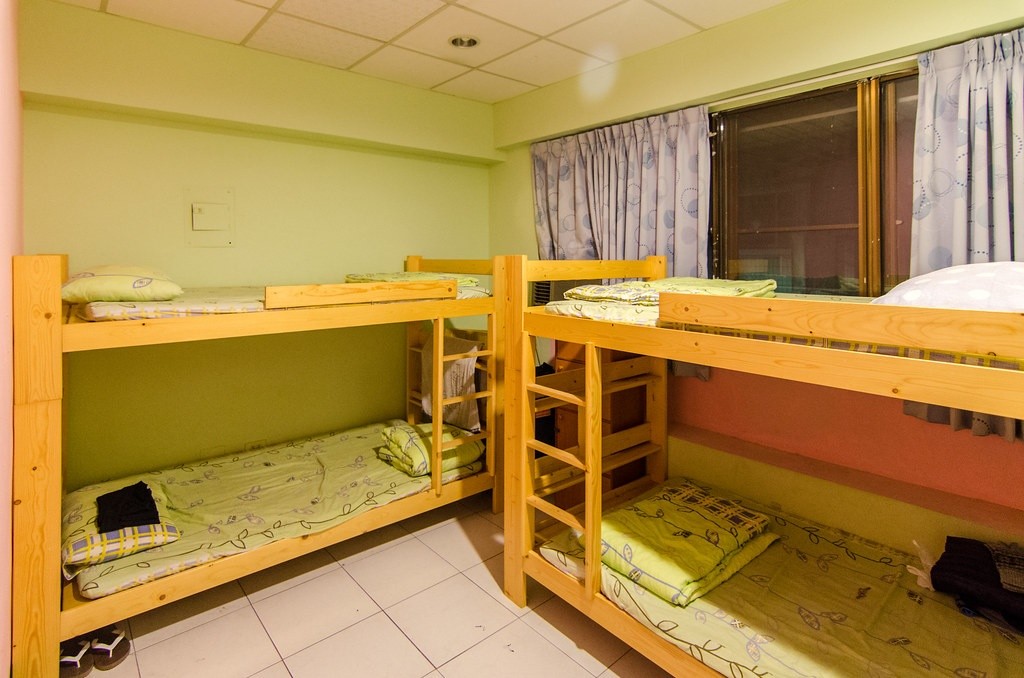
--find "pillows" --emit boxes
[62,482,180,581]
[61,264,184,303]
[868,261,1024,313]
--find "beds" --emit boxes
[504,254,1024,678]
[11,255,507,678]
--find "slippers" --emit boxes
[60,633,94,678]
[90,624,131,671]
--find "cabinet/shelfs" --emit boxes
[554,339,647,510]
